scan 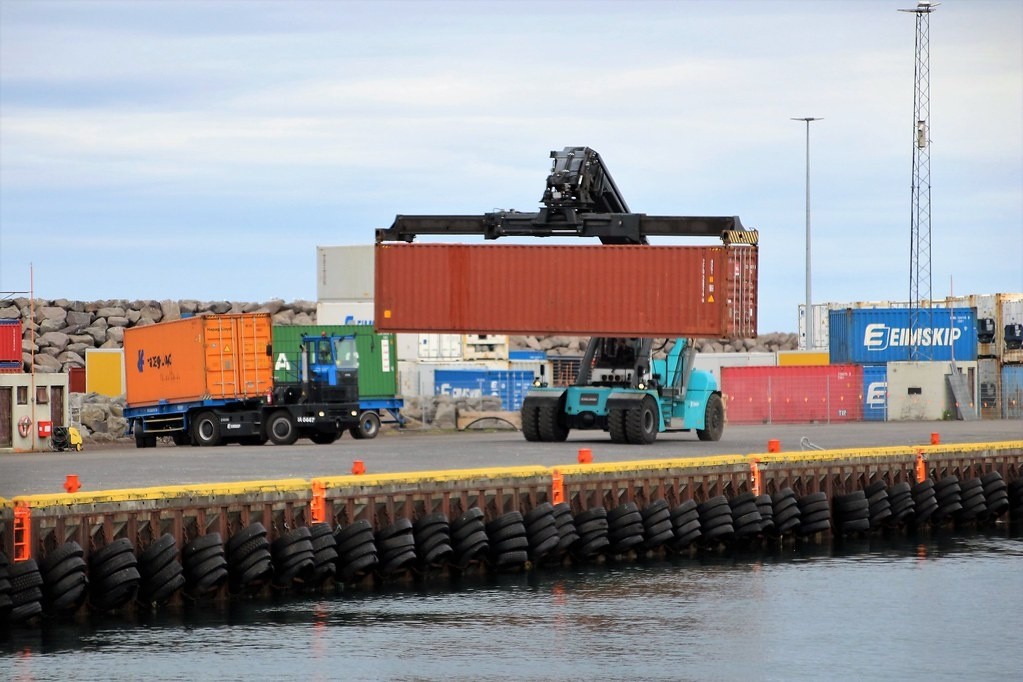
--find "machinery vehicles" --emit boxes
[375,146,761,444]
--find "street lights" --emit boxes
[909,2,937,359]
[789,117,826,349]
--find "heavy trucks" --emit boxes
[121,312,407,448]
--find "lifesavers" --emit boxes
[0,469,1023,622]
